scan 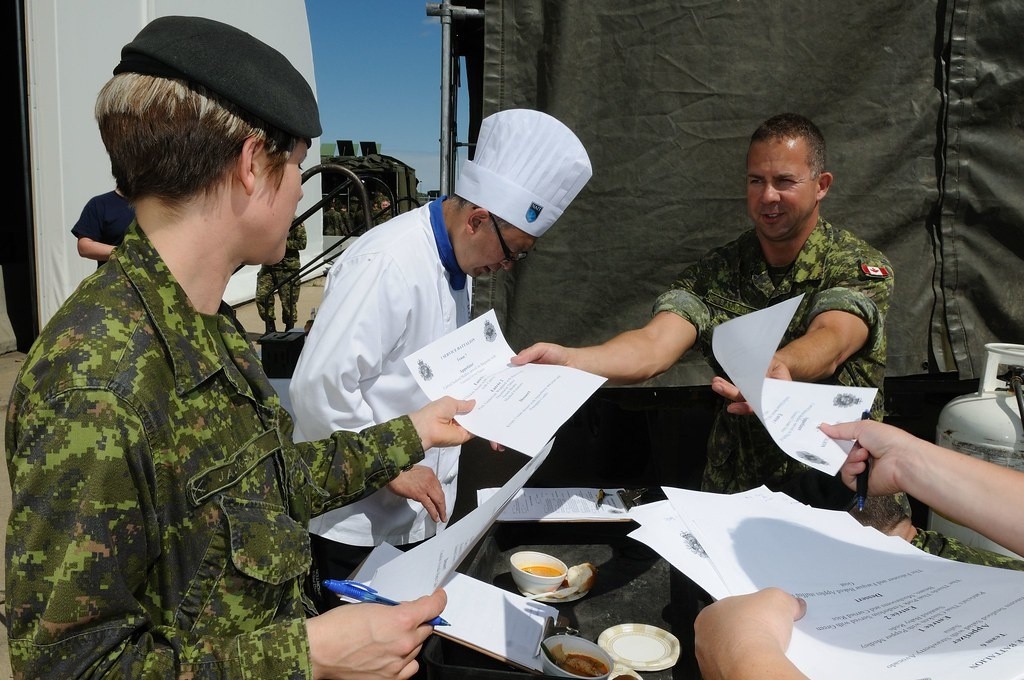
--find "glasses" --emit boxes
[471,205,528,263]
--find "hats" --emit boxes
[454,107,593,237]
[114,16,324,143]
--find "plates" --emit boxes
[516,585,589,602]
[597,623,681,672]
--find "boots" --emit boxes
[284,322,294,332]
[257,321,276,345]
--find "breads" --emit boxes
[567,562,597,593]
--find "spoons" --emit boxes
[522,586,579,603]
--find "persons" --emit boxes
[490,114,912,539]
[289,108,592,612]
[71,174,135,270]
[694,418,1024,680]
[255,215,307,332]
[0,17,476,680]
[323,189,395,237]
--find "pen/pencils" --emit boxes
[597,489,604,508]
[856,410,873,512]
[323,579,452,627]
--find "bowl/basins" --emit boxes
[540,634,614,680]
[509,551,568,595]
